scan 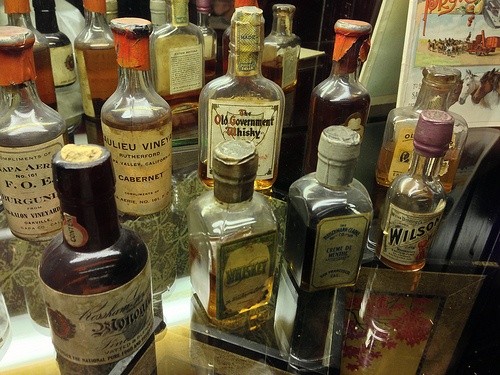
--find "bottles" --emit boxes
[196,1,222,80]
[374,111,448,270]
[108,17,175,216]
[0,25,71,242]
[4,0,58,112]
[301,19,373,176]
[31,0,76,88]
[148,1,206,101]
[197,7,284,191]
[289,126,374,291]
[38,144,154,366]
[184,140,288,327]
[222,0,260,78]
[376,65,467,193]
[74,0,118,118]
[262,4,300,93]
